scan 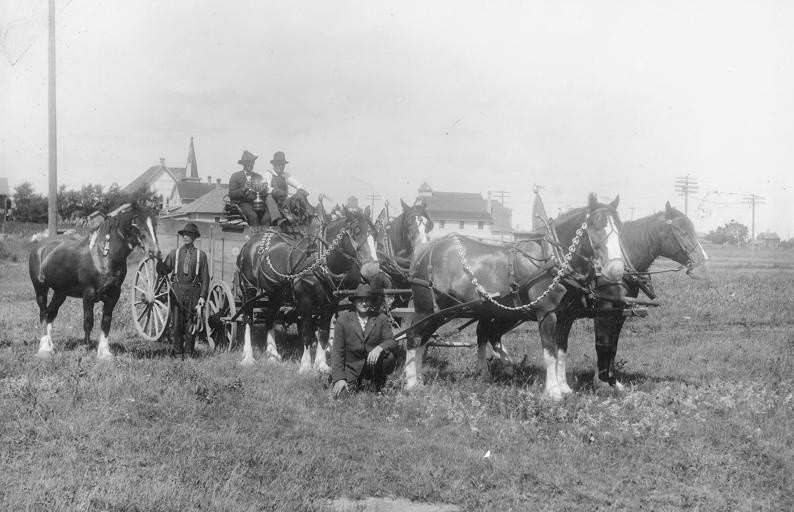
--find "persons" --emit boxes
[156,223,210,362]
[330,283,400,400]
[228,150,309,226]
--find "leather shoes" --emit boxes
[237,151,259,164]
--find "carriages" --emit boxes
[131,193,711,405]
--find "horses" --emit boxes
[324,197,433,358]
[28,197,164,363]
[402,192,625,403]
[236,203,380,379]
[475,200,711,394]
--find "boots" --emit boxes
[185,333,196,362]
[170,333,183,365]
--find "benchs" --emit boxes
[219,194,309,232]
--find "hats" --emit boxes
[270,152,289,164]
[348,285,379,302]
[178,223,200,237]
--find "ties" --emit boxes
[183,250,190,275]
